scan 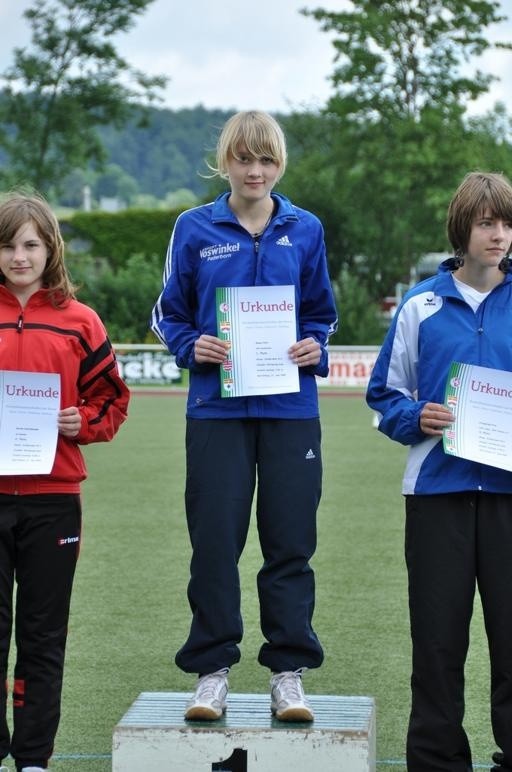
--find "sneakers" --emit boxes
[184,671,228,720]
[270,671,314,721]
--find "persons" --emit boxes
[151,108,341,724]
[2,187,130,771]
[361,166,508,771]
